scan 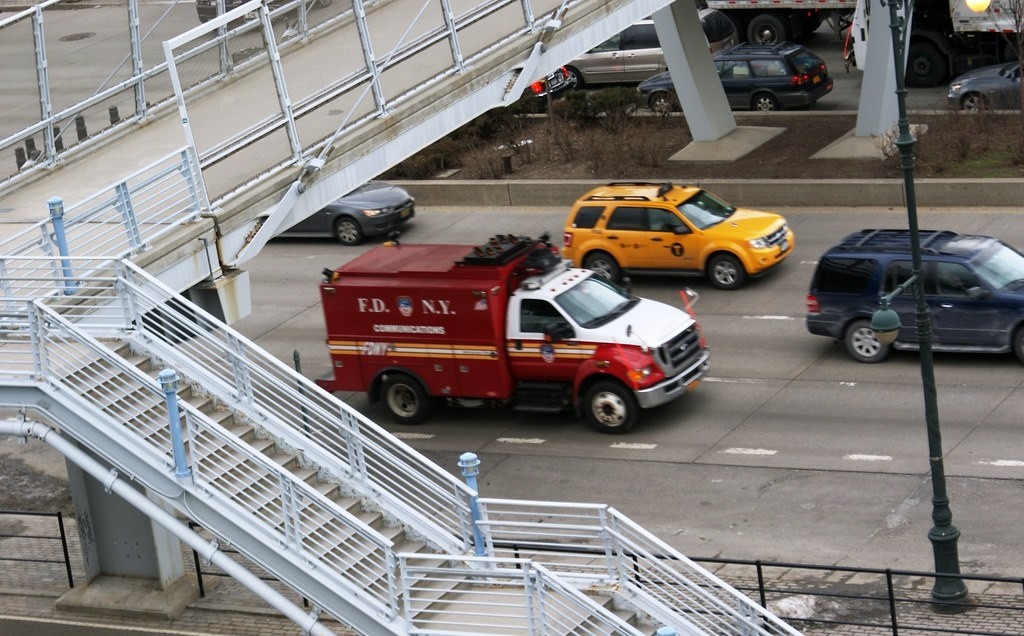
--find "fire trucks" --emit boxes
[315,232,710,435]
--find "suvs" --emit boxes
[564,6,739,90]
[561,182,796,290]
[633,42,834,111]
[806,229,1024,363]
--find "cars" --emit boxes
[195,0,332,31]
[947,60,1024,109]
[529,66,574,107]
[267,182,416,246]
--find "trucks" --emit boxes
[695,0,857,44]
[842,0,1024,88]
